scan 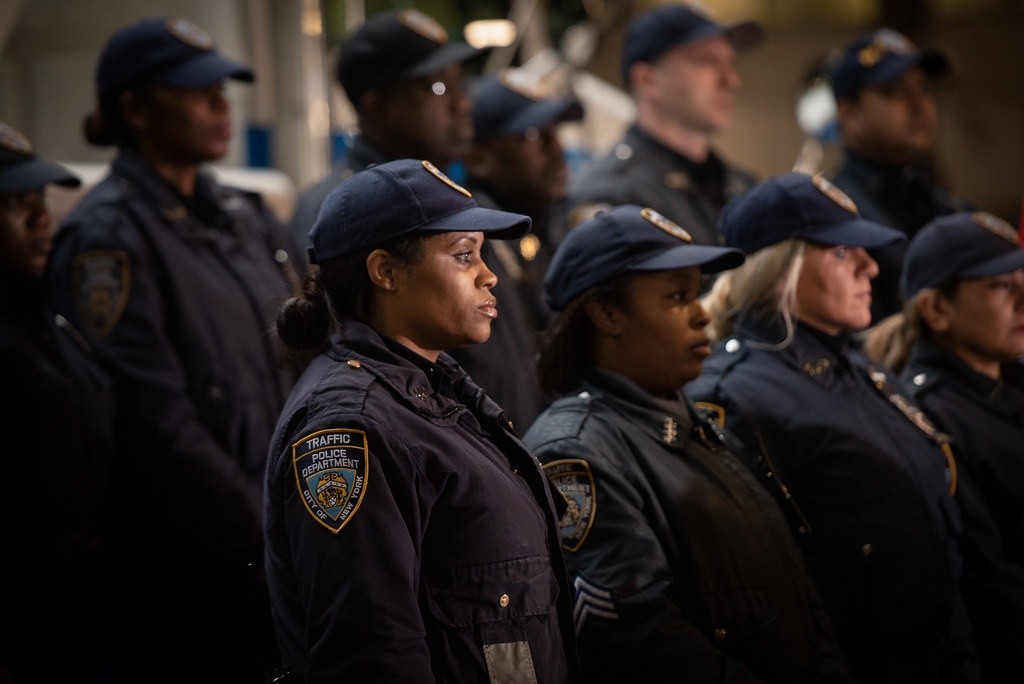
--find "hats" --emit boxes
[0,120,81,195]
[542,205,749,311]
[621,4,767,93]
[334,7,481,84]
[832,25,950,104]
[900,209,1024,303]
[305,157,533,271]
[721,171,908,249]
[96,14,257,96]
[473,66,584,138]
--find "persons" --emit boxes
[0,0,1024,683]
[261,158,581,684]
